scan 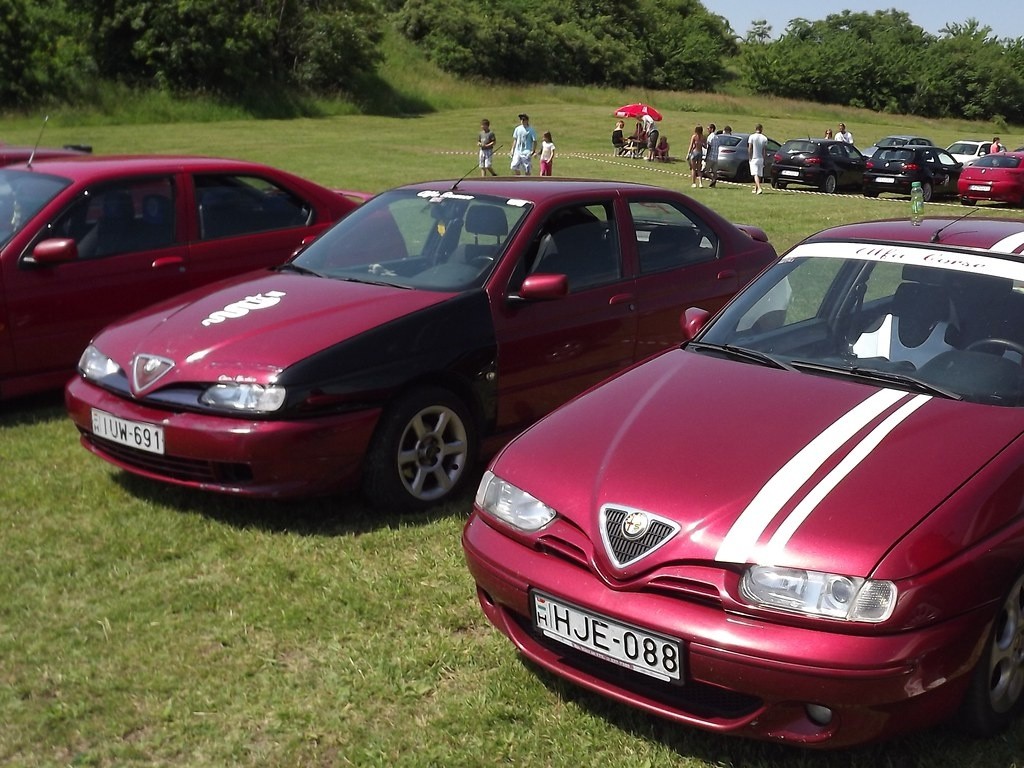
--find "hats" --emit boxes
[518,113,529,120]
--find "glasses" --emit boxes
[520,118,526,120]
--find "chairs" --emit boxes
[76,193,135,258]
[899,151,910,159]
[118,193,174,254]
[846,283,964,369]
[536,207,616,289]
[885,151,895,159]
[448,204,508,265]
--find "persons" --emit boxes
[509,113,538,175]
[632,122,648,159]
[612,120,631,157]
[477,119,497,176]
[824,128,833,139]
[990,136,1004,166]
[717,126,732,135]
[686,124,707,188]
[834,123,854,144]
[656,136,669,163]
[703,123,719,187]
[748,124,768,194]
[636,113,660,162]
[533,131,556,177]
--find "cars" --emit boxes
[0,143,94,167]
[0,156,409,401]
[66,178,777,510]
[462,215,1024,748]
[958,152,1024,206]
[700,134,783,183]
[771,138,870,194]
[857,135,935,163]
[927,140,1007,169]
[863,145,964,202]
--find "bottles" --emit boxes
[910,182,925,225]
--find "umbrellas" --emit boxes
[614,102,663,160]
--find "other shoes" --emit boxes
[691,184,697,187]
[757,188,762,195]
[709,180,716,188]
[698,184,703,187]
[752,189,757,193]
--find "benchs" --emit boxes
[639,224,715,277]
